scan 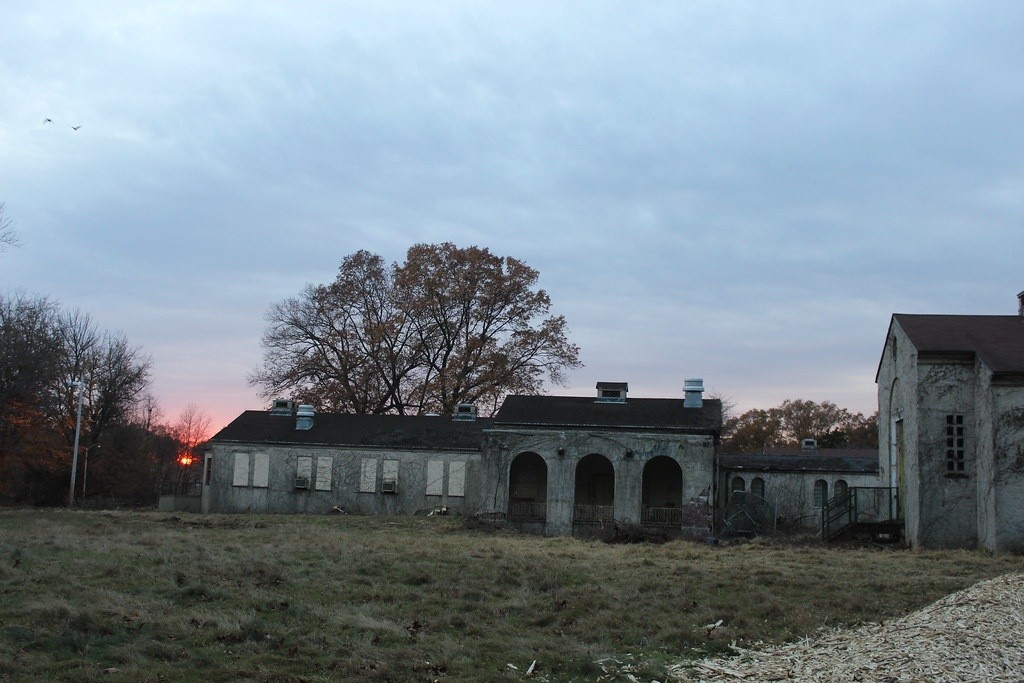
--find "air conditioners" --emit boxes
[381,481,396,493]
[294,478,309,488]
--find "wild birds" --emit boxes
[43,117,81,130]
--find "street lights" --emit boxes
[82,444,104,505]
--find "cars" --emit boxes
[414,506,464,520]
[478,511,504,528]
